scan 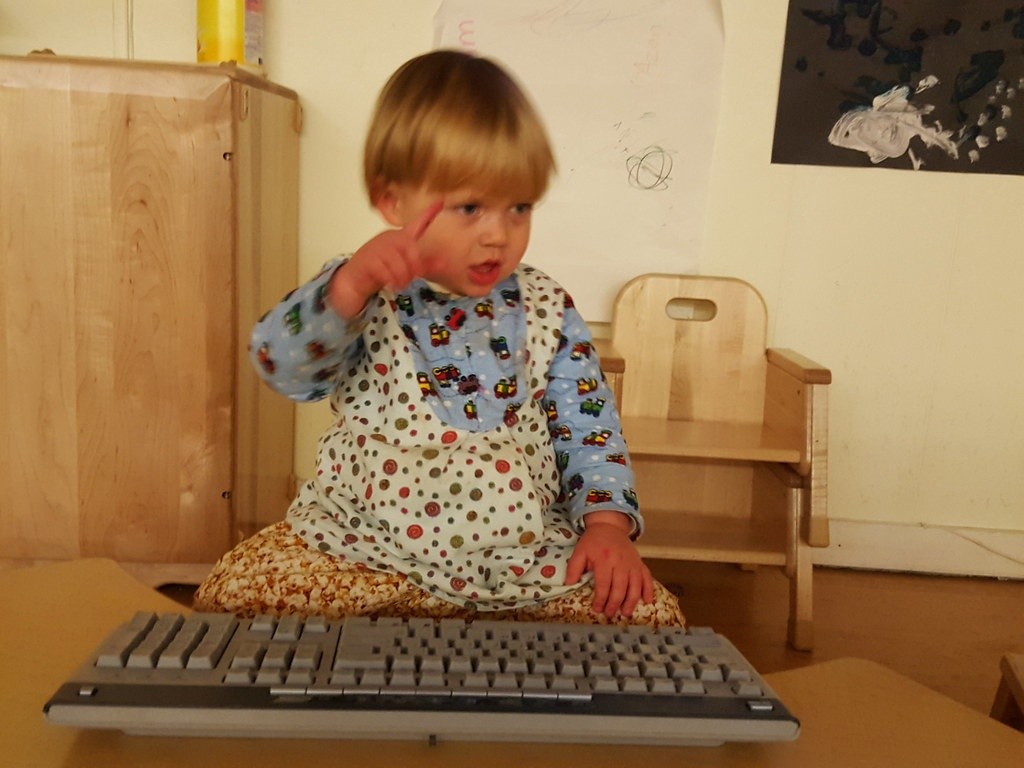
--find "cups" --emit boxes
[198,0,263,63]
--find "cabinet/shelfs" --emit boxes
[0,52,300,564]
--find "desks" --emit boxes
[0,555,1024,768]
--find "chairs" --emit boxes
[592,272,832,655]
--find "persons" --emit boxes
[193,49,688,629]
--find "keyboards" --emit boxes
[41,611,802,747]
